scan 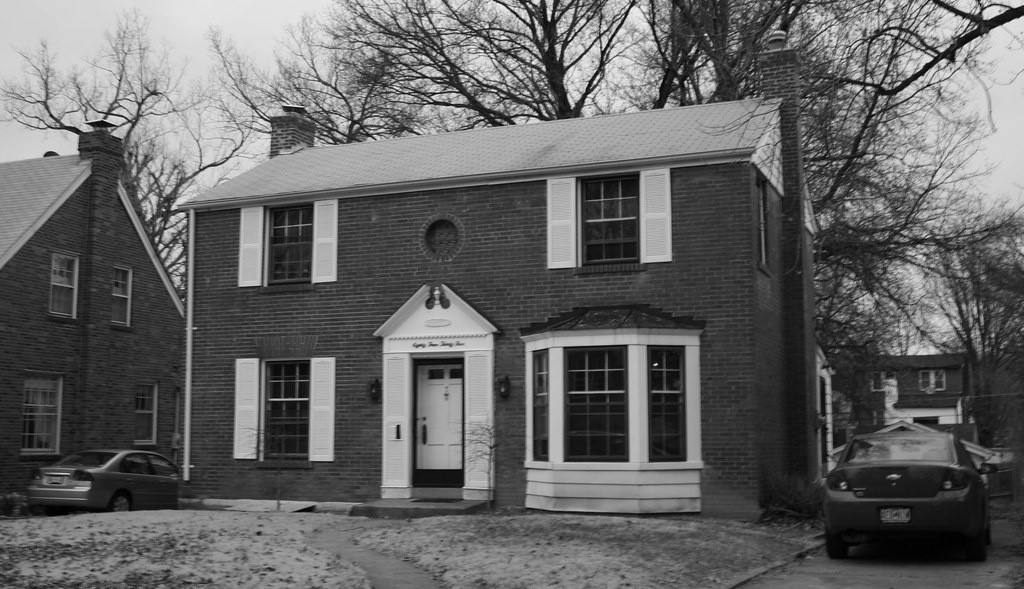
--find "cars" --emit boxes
[27,449,181,511]
[819,428,998,563]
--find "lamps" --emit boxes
[367,378,381,399]
[495,376,510,397]
